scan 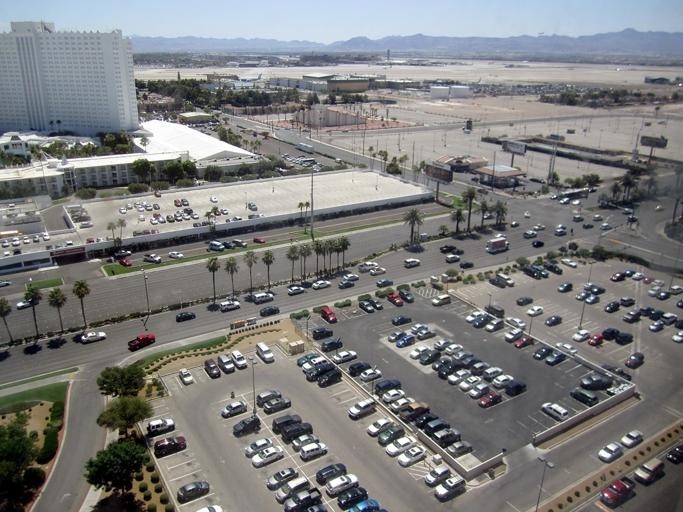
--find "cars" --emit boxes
[376,279,393,288]
[3,233,74,257]
[557,270,683,346]
[544,315,561,326]
[266,463,379,512]
[666,445,683,463]
[287,274,360,295]
[80,222,116,244]
[511,187,664,247]
[176,311,195,322]
[348,378,472,467]
[598,429,644,463]
[177,482,209,503]
[370,267,387,275]
[17,299,39,309]
[359,298,382,312]
[222,390,329,469]
[484,213,494,219]
[0,279,11,287]
[115,250,132,267]
[169,252,183,259]
[282,154,324,171]
[119,192,264,236]
[579,374,612,389]
[81,331,107,344]
[496,234,506,237]
[388,316,435,348]
[179,368,193,384]
[410,339,527,408]
[441,245,473,268]
[253,237,265,243]
[260,306,280,316]
[624,352,643,369]
[197,504,222,512]
[561,258,577,268]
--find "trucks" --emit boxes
[321,307,337,323]
[603,364,632,381]
[486,237,510,254]
[523,261,562,279]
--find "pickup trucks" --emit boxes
[600,476,635,506]
[358,261,378,272]
[128,334,155,350]
[143,253,161,264]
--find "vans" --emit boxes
[533,342,578,366]
[68,205,91,223]
[220,293,273,312]
[570,387,599,407]
[432,294,450,306]
[256,342,274,362]
[297,327,382,387]
[425,467,466,501]
[388,288,414,306]
[488,272,514,287]
[466,299,543,347]
[209,239,247,251]
[541,403,568,421]
[634,458,664,484]
[205,350,247,378]
[405,258,421,268]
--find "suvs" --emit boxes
[148,419,175,437]
[155,435,186,458]
